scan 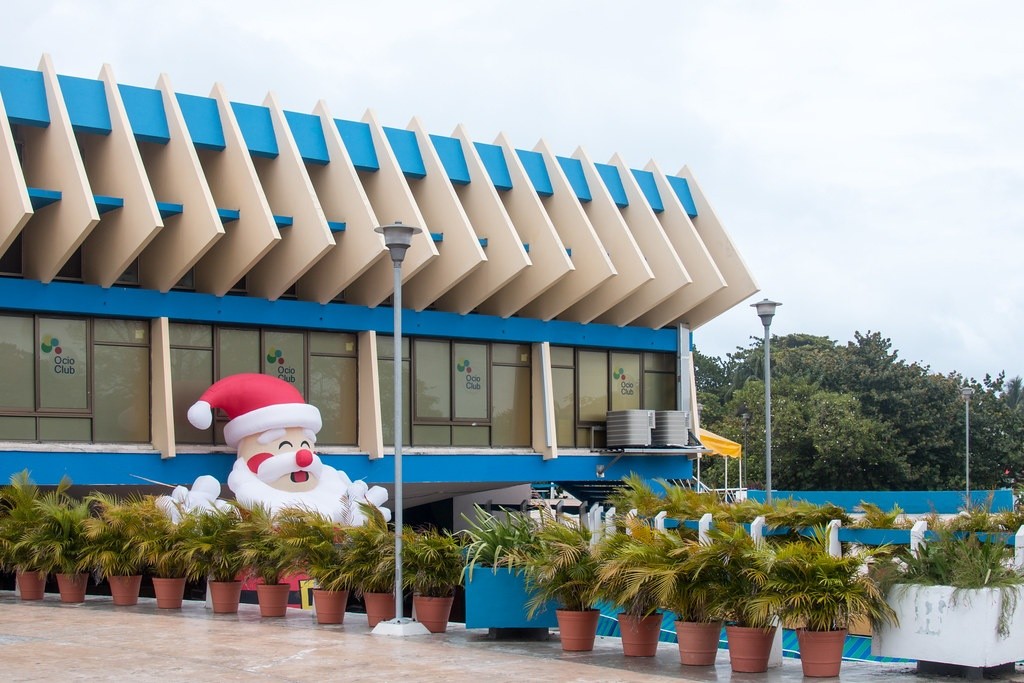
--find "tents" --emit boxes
[697,428,741,503]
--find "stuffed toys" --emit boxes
[154,373,392,541]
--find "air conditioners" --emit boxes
[0,461,1024,677]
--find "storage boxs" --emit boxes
[606,410,655,447]
[650,410,687,447]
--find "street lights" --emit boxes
[960,386,972,510]
[749,297,783,506]
[372,220,432,636]
